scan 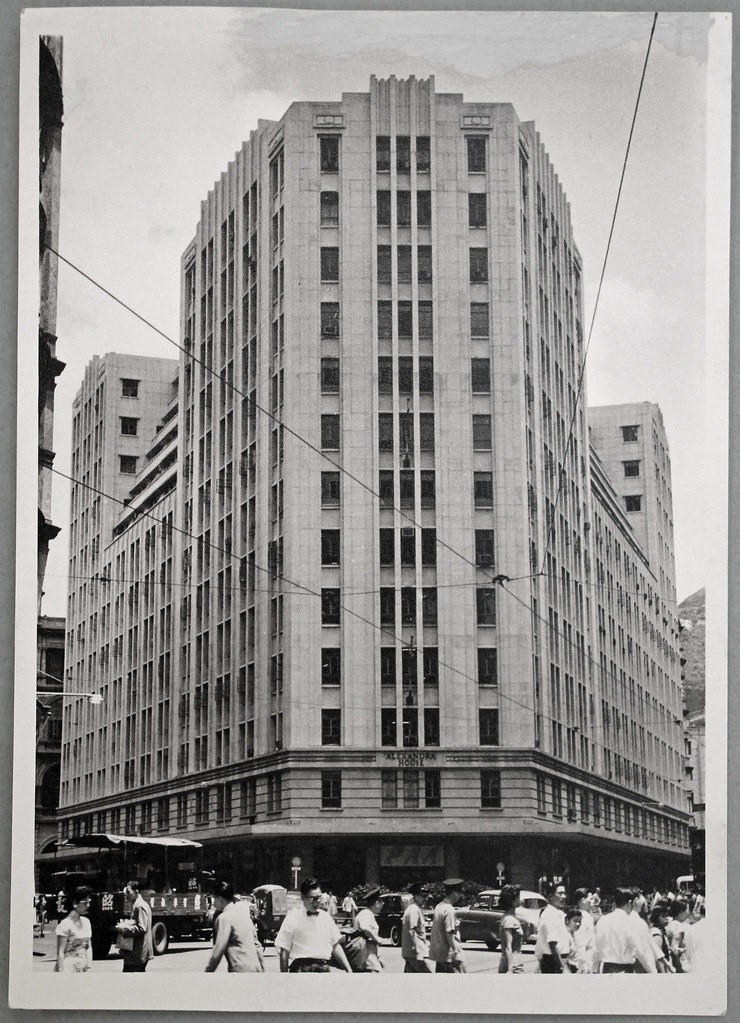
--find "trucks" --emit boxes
[45,833,254,956]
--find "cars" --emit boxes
[259,891,303,917]
[454,889,550,949]
[375,892,435,946]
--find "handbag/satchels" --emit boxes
[115,932,135,951]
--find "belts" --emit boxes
[561,954,568,958]
[312,959,331,965]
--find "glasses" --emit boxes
[304,894,322,903]
[81,899,93,905]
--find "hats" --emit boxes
[362,887,384,903]
[442,878,465,888]
[407,882,430,894]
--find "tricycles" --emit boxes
[249,884,288,955]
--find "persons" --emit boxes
[342,892,358,927]
[353,886,391,973]
[401,881,432,973]
[535,881,603,975]
[37,894,47,938]
[52,886,93,973]
[429,878,465,973]
[115,881,155,973]
[204,881,266,972]
[591,884,705,973]
[249,898,258,928]
[205,906,213,941]
[498,884,525,974]
[324,888,338,917]
[274,878,353,973]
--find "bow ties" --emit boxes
[306,910,320,916]
